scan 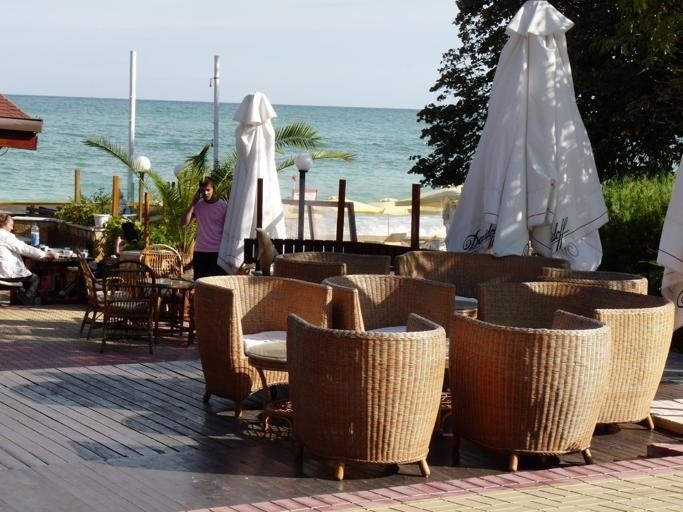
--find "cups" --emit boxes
[41,245,88,259]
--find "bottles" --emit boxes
[30,223,39,248]
[521,241,537,256]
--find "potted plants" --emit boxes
[90,187,114,231]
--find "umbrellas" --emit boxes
[654,153,683,335]
[443,0,609,274]
[218,91,288,277]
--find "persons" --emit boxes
[88,222,145,278]
[180,176,226,281]
[0,212,55,306]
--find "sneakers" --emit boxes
[19,283,81,305]
